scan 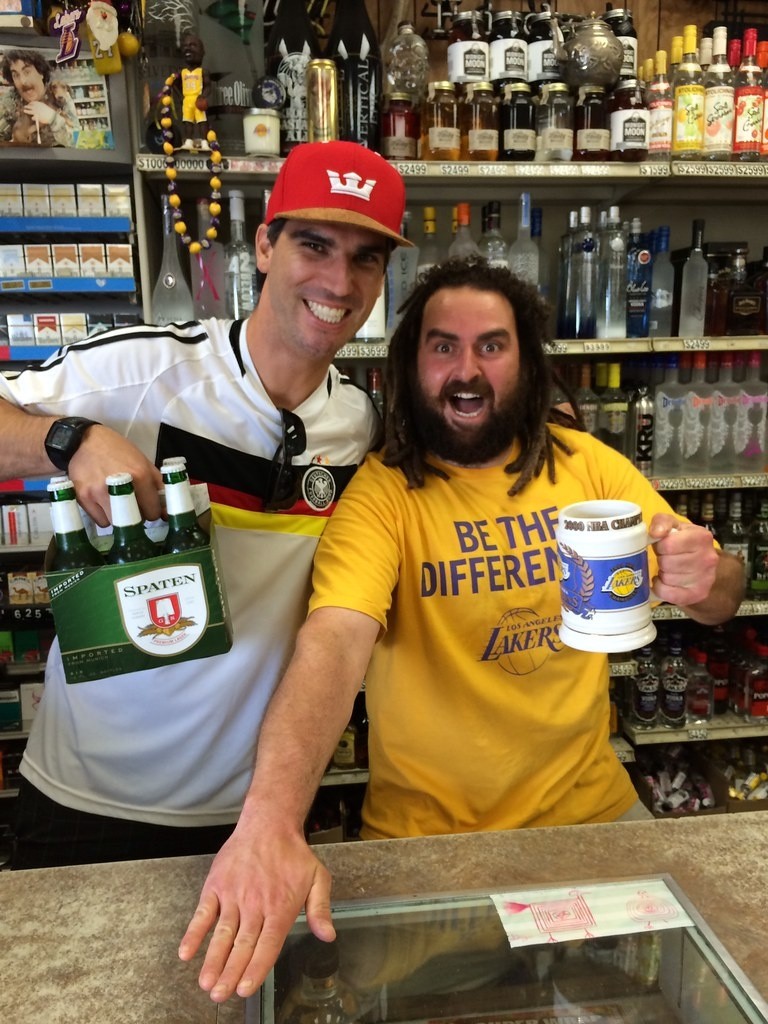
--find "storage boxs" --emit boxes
[0,571,51,605]
[630,745,768,819]
[0,183,132,221]
[0,313,140,346]
[308,794,348,845]
[0,683,45,733]
[0,244,133,277]
[45,482,233,683]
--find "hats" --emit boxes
[265,138,415,248]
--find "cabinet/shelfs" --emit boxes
[0,28,162,799]
[130,150,768,787]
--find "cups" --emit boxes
[557,500,679,653]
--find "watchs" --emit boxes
[44,417,103,472]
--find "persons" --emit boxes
[0,142,412,872]
[1,50,78,147]
[178,264,746,1002]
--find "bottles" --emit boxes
[668,490,768,604]
[150,189,277,326]
[377,191,768,340]
[613,620,768,731]
[377,9,768,166]
[366,361,389,425]
[273,946,360,1024]
[242,107,282,159]
[44,457,210,573]
[545,350,767,485]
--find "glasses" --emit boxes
[261,407,307,513]
